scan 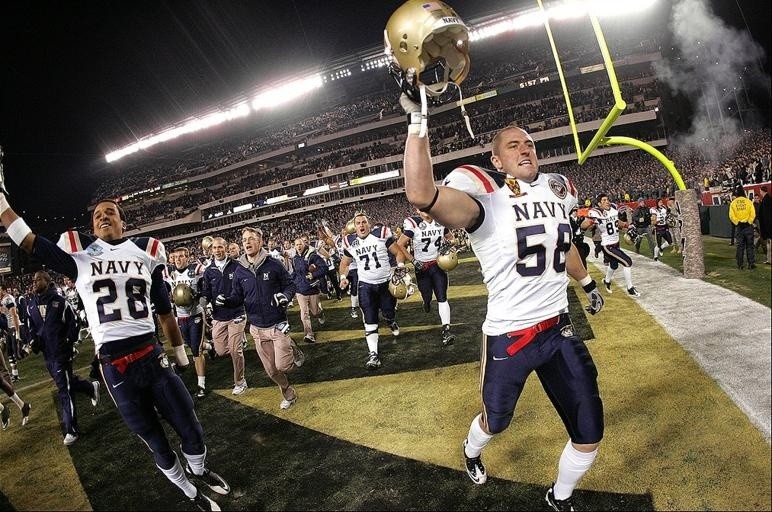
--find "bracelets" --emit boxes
[174,344,190,366]
[1,192,10,215]
[580,273,591,286]
[407,123,431,137]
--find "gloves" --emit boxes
[172,343,190,370]
[270,291,289,308]
[410,257,424,271]
[580,279,606,316]
[397,85,428,136]
[435,237,452,256]
[214,291,232,308]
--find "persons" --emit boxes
[392,63,604,510]
[1,148,230,511]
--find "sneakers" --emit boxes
[288,335,306,369]
[278,394,299,410]
[302,331,316,343]
[238,332,250,352]
[181,483,222,511]
[287,291,360,319]
[363,351,383,372]
[625,284,642,298]
[544,480,575,512]
[206,338,219,361]
[387,316,401,336]
[440,322,457,348]
[461,437,489,485]
[592,242,772,271]
[601,277,614,295]
[196,384,207,399]
[231,380,249,396]
[316,304,327,329]
[184,460,233,497]
[0,324,102,446]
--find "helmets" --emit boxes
[172,283,195,307]
[382,1,473,99]
[434,248,459,273]
[622,231,636,246]
[344,217,356,235]
[387,267,416,300]
[201,235,215,251]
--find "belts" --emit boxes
[109,339,156,375]
